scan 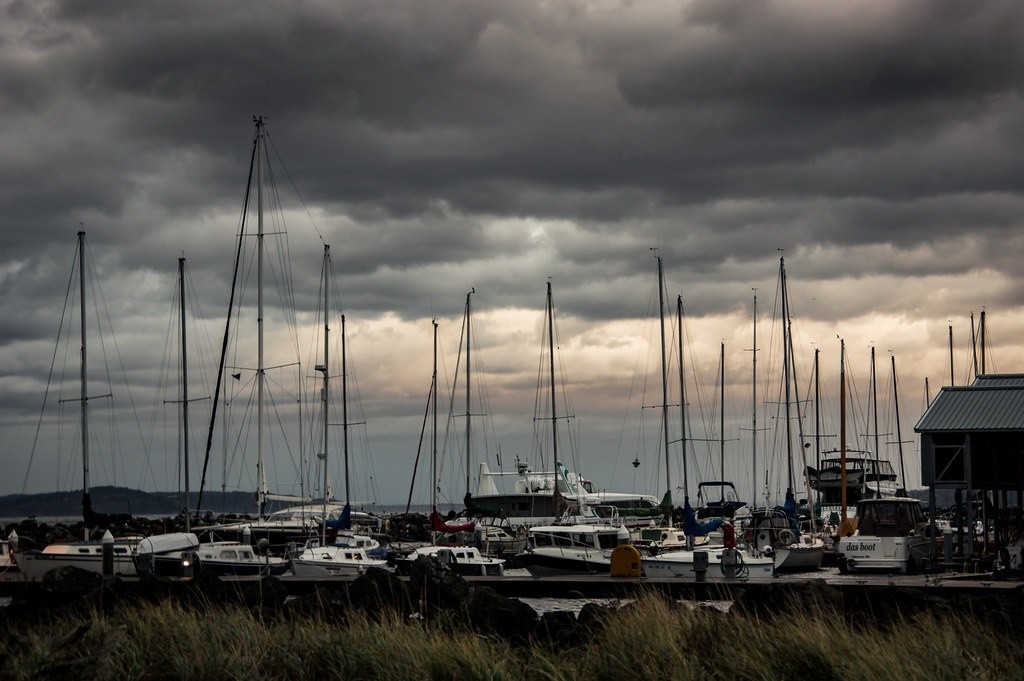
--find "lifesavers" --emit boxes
[778,528,795,544]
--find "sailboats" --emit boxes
[0,118,995,589]
